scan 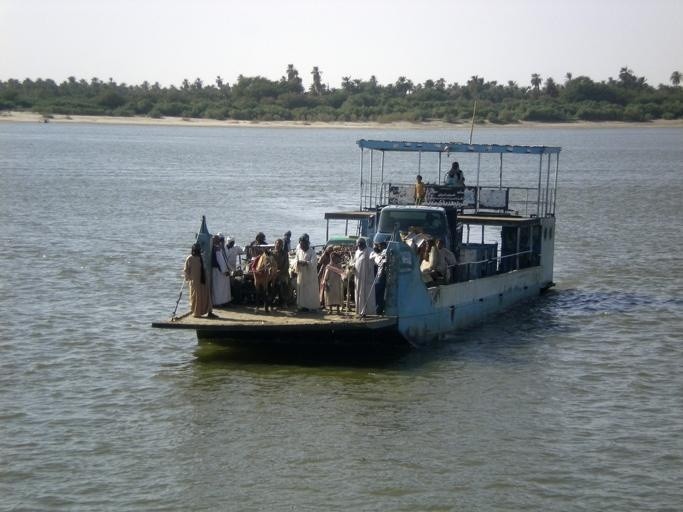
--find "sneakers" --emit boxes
[254,301,344,315]
[208,313,217,318]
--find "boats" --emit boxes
[150,98,563,346]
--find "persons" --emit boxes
[182,230,456,318]
[445,161,464,185]
[413,175,427,206]
